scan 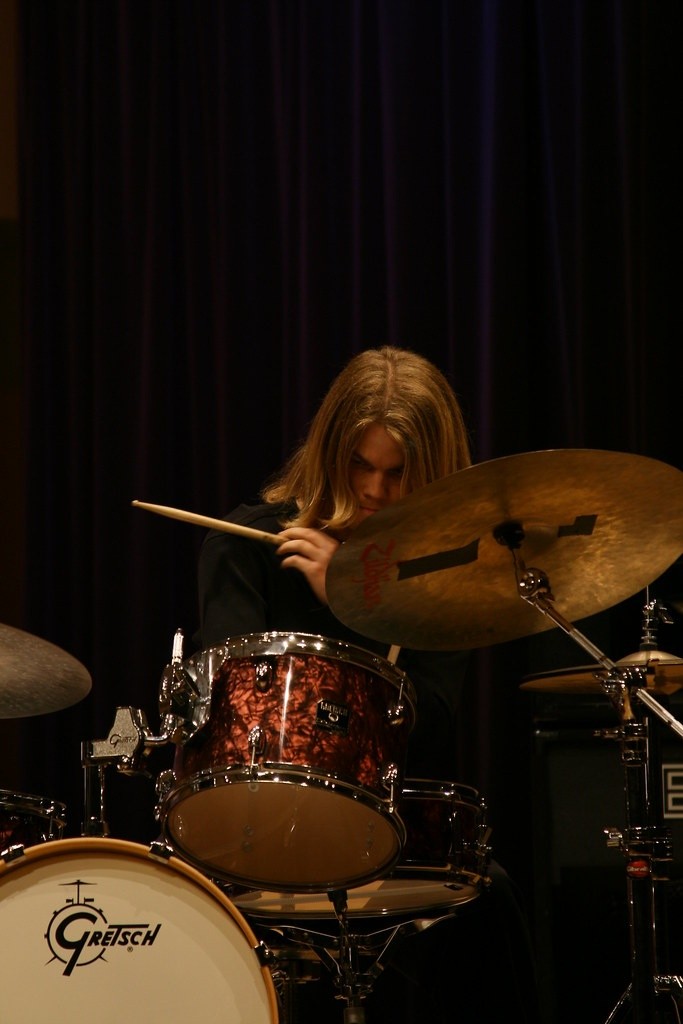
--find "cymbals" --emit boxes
[520,650,683,696]
[324,446,682,657]
[0,624,93,719]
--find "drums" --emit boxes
[221,775,492,924]
[0,790,69,858]
[155,627,404,895]
[0,836,274,1024]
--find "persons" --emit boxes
[196,344,477,780]
